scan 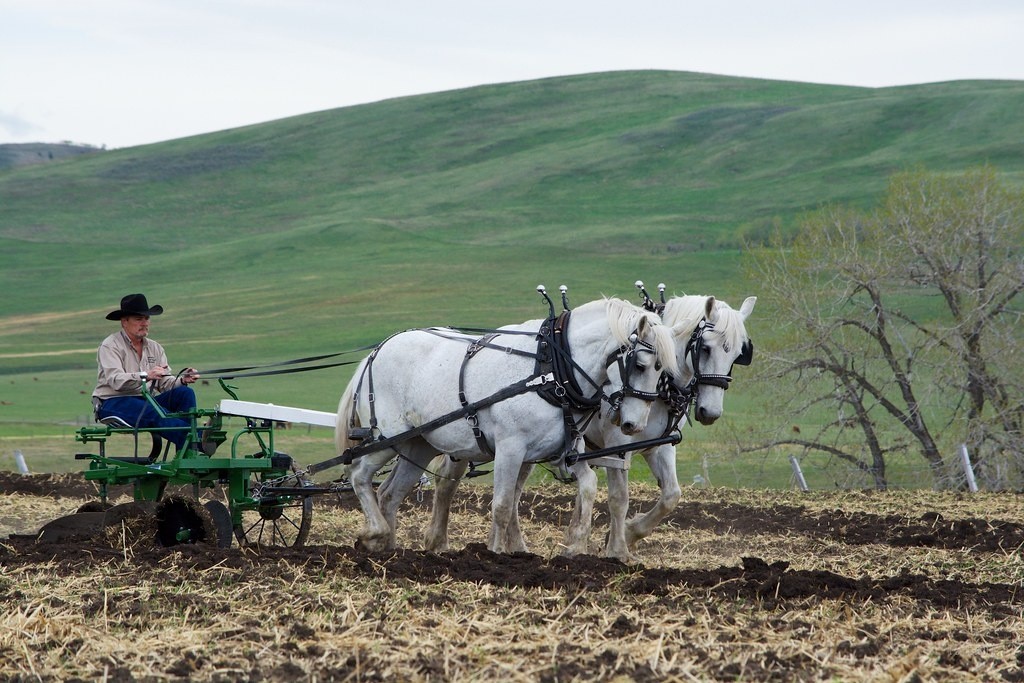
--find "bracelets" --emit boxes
[181,377,185,385]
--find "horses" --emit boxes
[331,293,757,559]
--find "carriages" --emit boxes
[74,270,760,562]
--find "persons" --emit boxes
[92,293,222,455]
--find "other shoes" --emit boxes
[202,417,218,456]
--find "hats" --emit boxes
[106,293,163,321]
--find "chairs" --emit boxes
[92,396,140,434]
